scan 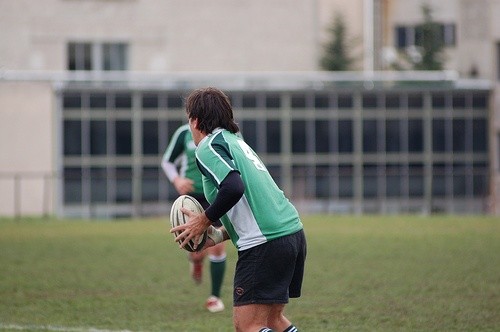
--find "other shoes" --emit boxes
[206,295,224,313]
[192,263,201,282]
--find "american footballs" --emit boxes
[169,193,208,254]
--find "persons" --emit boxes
[170,87,307,332]
[160,124,244,312]
